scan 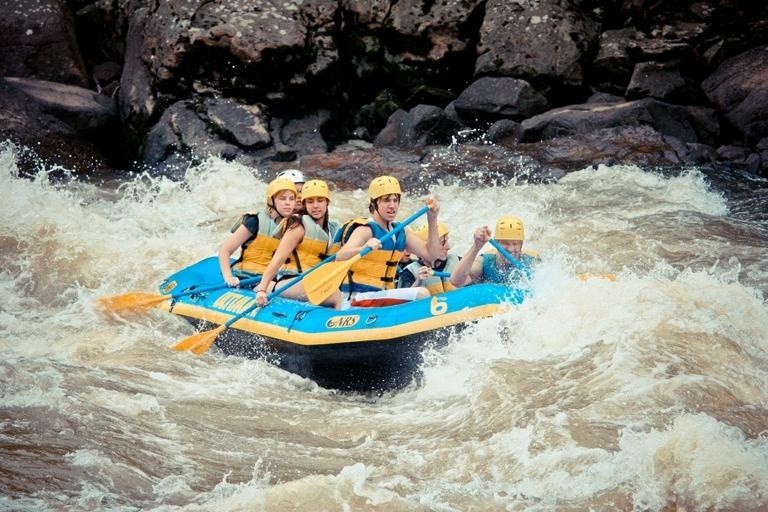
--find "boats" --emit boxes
[157,254,533,395]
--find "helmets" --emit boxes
[277,169,306,183]
[368,175,402,204]
[301,180,329,201]
[267,179,297,200]
[494,217,525,241]
[415,223,450,243]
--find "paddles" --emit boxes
[303,196,436,305]
[170,253,337,357]
[91,275,262,316]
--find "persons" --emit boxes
[219,169,463,309]
[450,215,547,287]
